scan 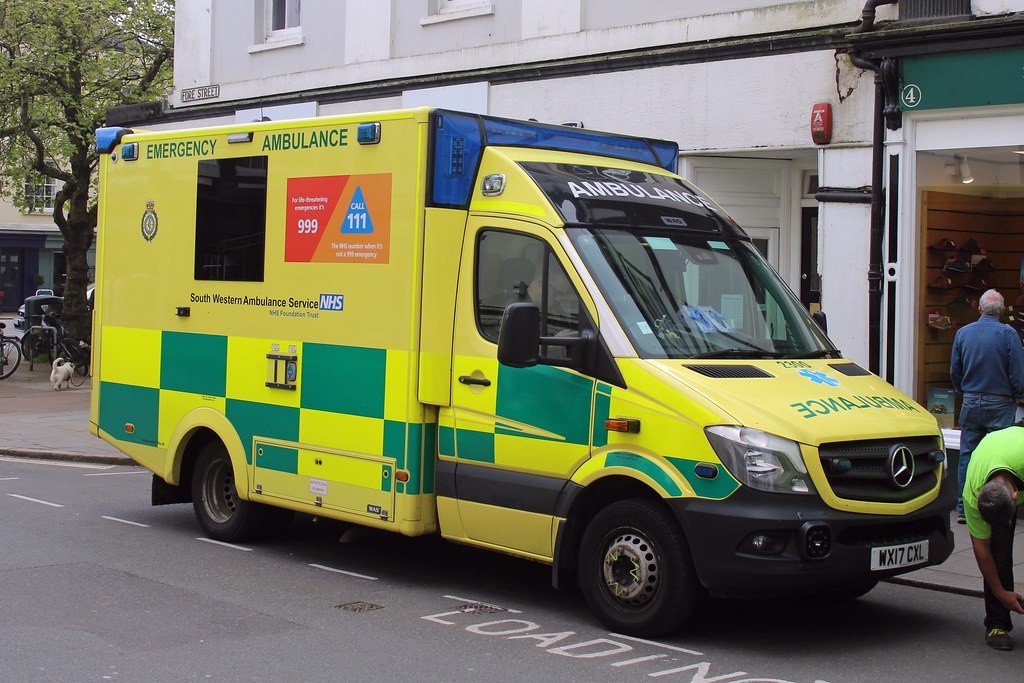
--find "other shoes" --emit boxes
[957,515,966,523]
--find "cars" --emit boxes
[14,295,65,333]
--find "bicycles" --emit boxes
[22,308,93,380]
[0,316,22,383]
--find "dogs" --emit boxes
[50,357,75,391]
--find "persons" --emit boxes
[487,259,577,359]
[950,288,1024,523]
[961,426,1024,649]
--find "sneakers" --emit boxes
[985,628,1012,650]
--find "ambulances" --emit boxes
[85,106,957,633]
